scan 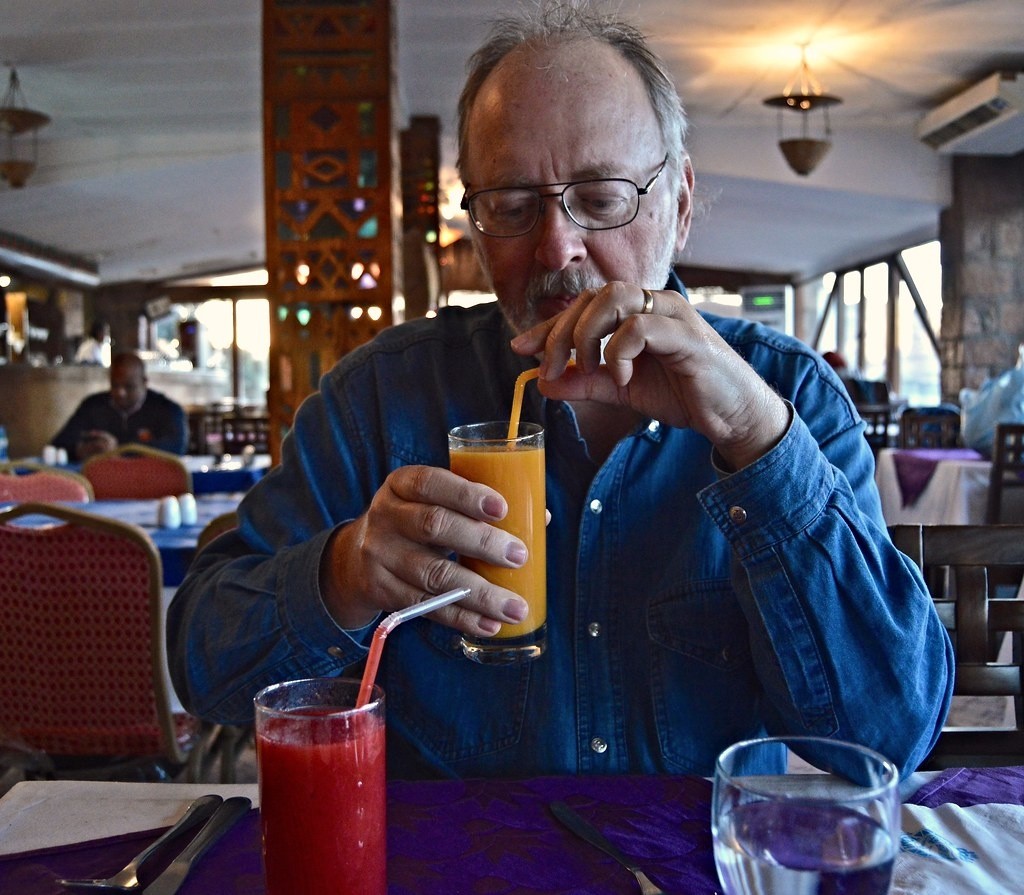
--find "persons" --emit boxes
[165,0,956,779]
[50,352,190,462]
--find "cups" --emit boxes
[159,495,182,529]
[42,446,69,466]
[178,493,197,525]
[254,677,387,895]
[449,422,548,665]
[711,736,901,895]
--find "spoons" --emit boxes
[56,794,224,890]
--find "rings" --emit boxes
[642,289,654,314]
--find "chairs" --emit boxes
[0,405,1024,795]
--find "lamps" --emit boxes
[764,41,844,174]
[0,67,51,188]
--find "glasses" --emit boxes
[459,153,670,237]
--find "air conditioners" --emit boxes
[918,73,1024,154]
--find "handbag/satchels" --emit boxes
[957,366,1024,469]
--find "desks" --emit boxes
[21,454,272,494]
[0,494,248,587]
[0,768,1024,895]
[875,447,1024,526]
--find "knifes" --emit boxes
[141,796,251,895]
